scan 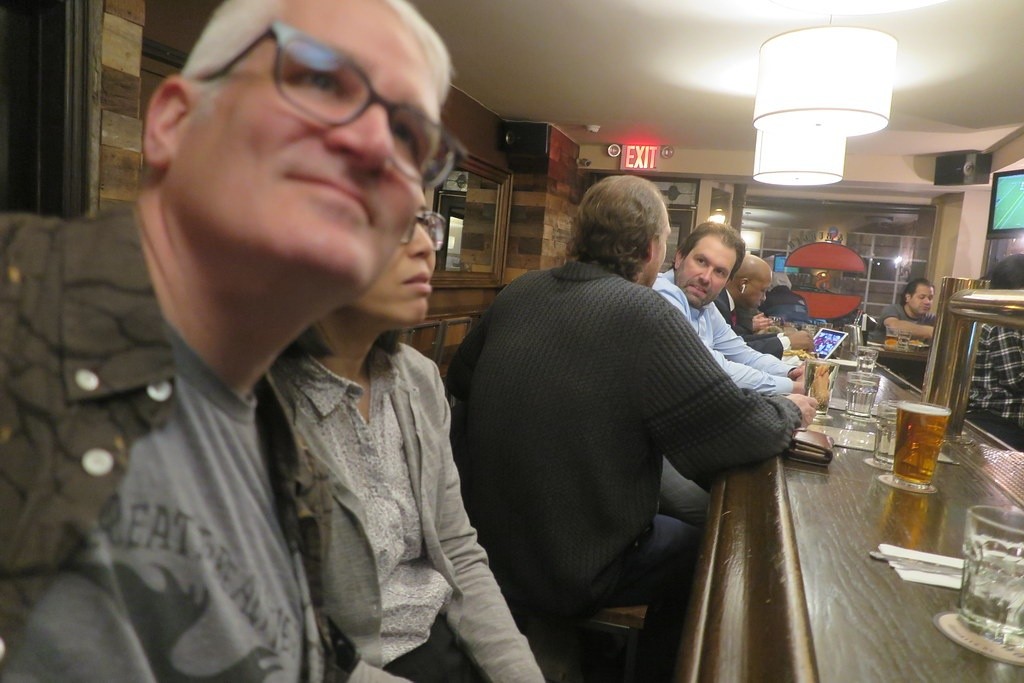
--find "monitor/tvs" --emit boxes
[986,169,1024,239]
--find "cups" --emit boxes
[885,326,897,350]
[803,359,839,412]
[857,345,879,374]
[892,400,952,489]
[898,327,911,350]
[873,400,905,466]
[765,314,833,338]
[842,325,863,356]
[846,370,880,419]
[959,506,1023,656]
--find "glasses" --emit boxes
[418,210,447,250]
[191,21,467,187]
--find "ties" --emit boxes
[731,309,737,329]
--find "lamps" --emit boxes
[753,14,897,186]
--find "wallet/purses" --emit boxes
[785,427,833,465]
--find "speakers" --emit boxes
[934,153,992,186]
[497,119,549,155]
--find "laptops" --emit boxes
[814,328,848,360]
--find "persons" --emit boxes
[965,253,1024,451]
[715,253,815,360]
[649,220,806,522]
[445,177,821,683]
[265,189,551,683]
[759,269,809,322]
[1,0,460,683]
[872,277,936,341]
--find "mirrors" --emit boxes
[424,151,513,288]
